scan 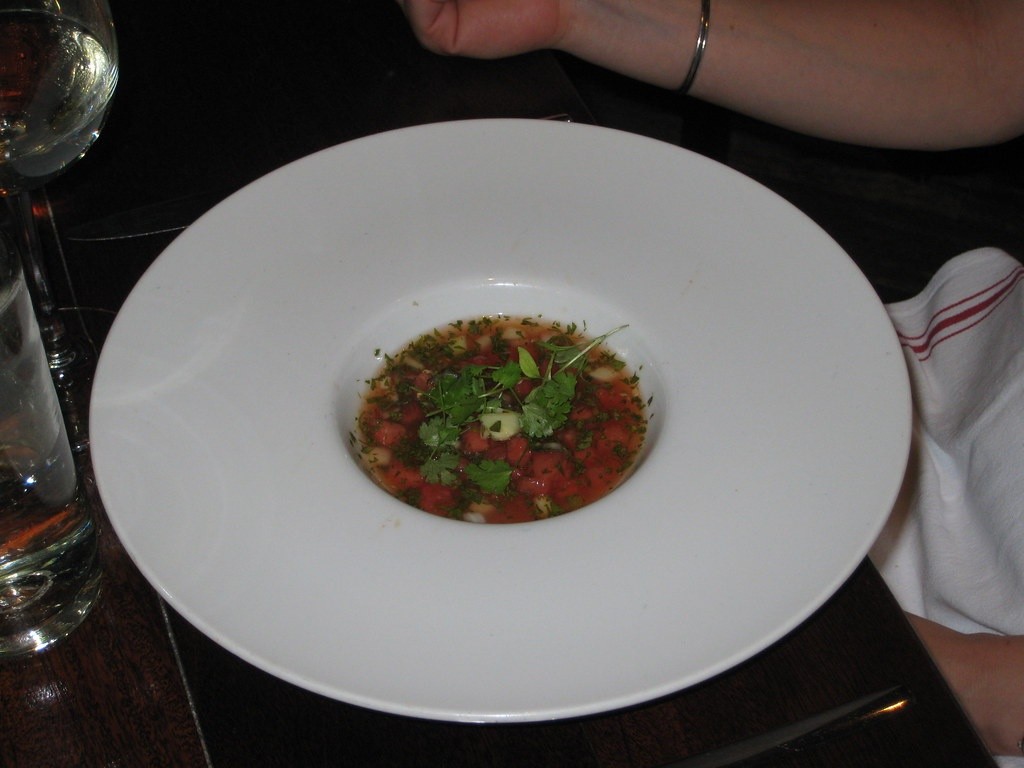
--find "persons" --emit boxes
[401,0,1024,757]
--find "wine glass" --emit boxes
[0,0,118,455]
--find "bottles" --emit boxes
[0,159,105,662]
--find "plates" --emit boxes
[90,119,913,727]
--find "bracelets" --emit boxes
[678,0,710,94]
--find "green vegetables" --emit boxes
[345,312,654,523]
